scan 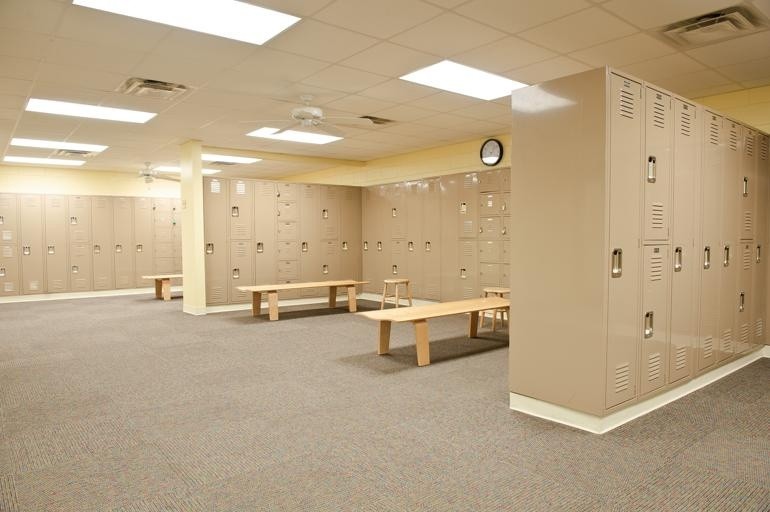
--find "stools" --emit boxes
[479,286,510,336]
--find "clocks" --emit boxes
[479,139,503,166]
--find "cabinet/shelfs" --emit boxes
[0,188,183,303]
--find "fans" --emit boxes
[241,94,373,138]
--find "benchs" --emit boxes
[356,297,510,368]
